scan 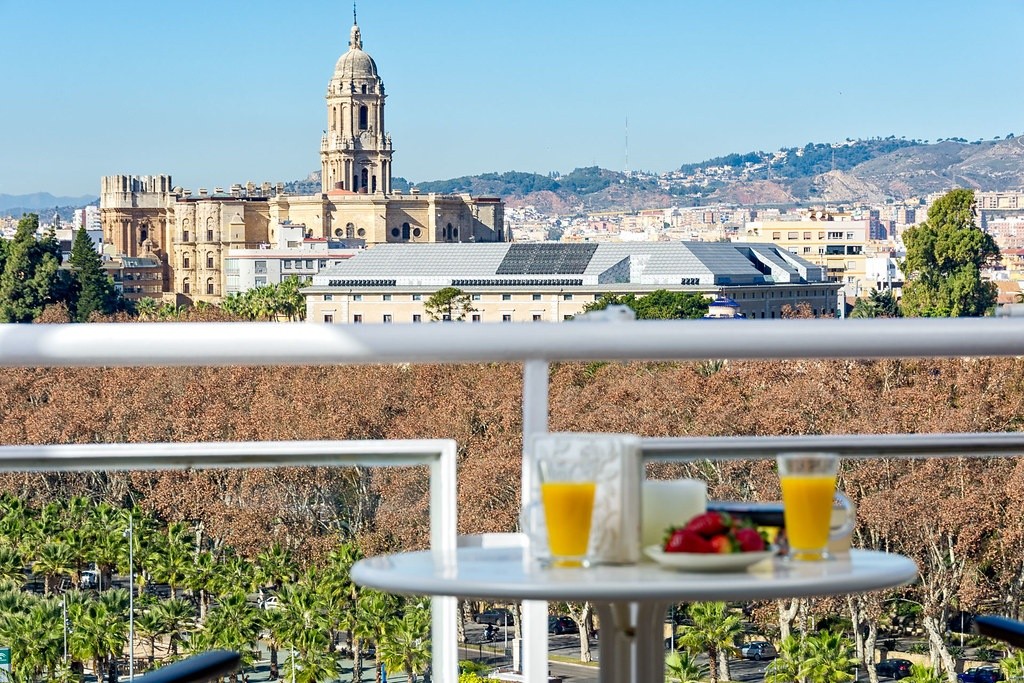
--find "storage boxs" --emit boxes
[519,432,641,568]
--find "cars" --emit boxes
[875,658,914,680]
[591,629,600,640]
[21,582,46,595]
[259,597,280,610]
[736,641,777,661]
[665,636,683,650]
[473,608,514,626]
[548,616,576,635]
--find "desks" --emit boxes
[347,545,919,683]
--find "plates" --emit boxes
[643,541,779,570]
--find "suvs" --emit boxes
[245,588,272,604]
[957,666,1004,683]
[81,571,96,589]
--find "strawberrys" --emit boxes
[664,511,765,553]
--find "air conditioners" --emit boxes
[260,244,271,249]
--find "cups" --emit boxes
[536,457,600,568]
[775,453,838,561]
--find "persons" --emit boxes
[485,622,493,635]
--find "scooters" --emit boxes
[481,627,499,641]
